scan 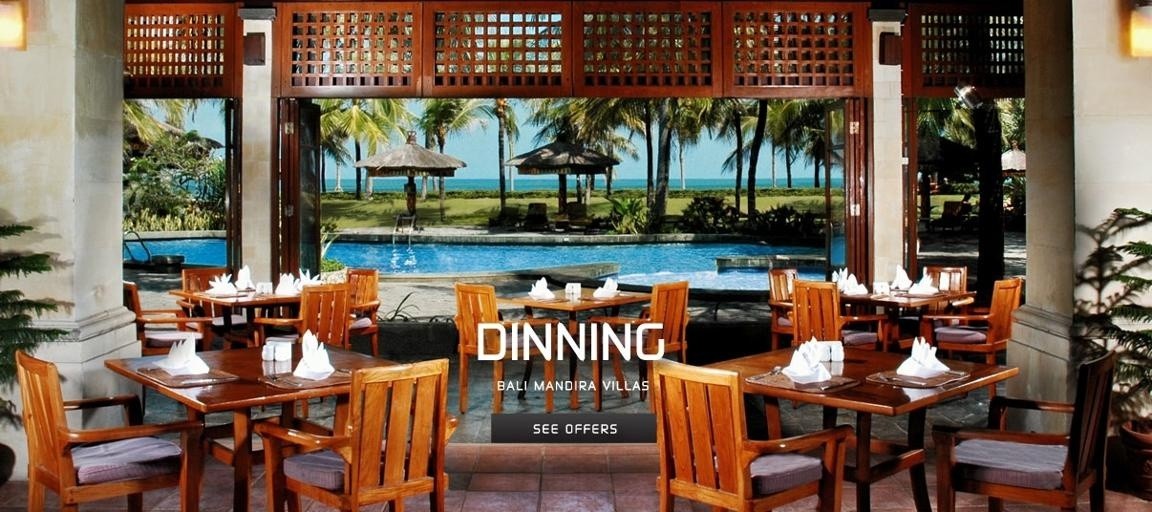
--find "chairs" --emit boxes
[933,350,1118,512]
[652,357,853,512]
[769,266,1022,398]
[589,281,689,410]
[123,267,382,354]
[933,201,971,231]
[252,357,451,512]
[490,207,517,230]
[518,204,549,230]
[12,348,204,512]
[455,283,558,414]
[556,201,591,233]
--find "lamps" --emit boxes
[1130,5,1152,57]
[0,1,24,48]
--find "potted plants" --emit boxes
[0,225,74,486]
[1071,207,1152,502]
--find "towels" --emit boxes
[528,276,555,301]
[830,264,939,297]
[779,336,832,386]
[152,333,211,378]
[896,335,951,379]
[205,264,323,296]
[292,328,336,382]
[591,277,622,299]
